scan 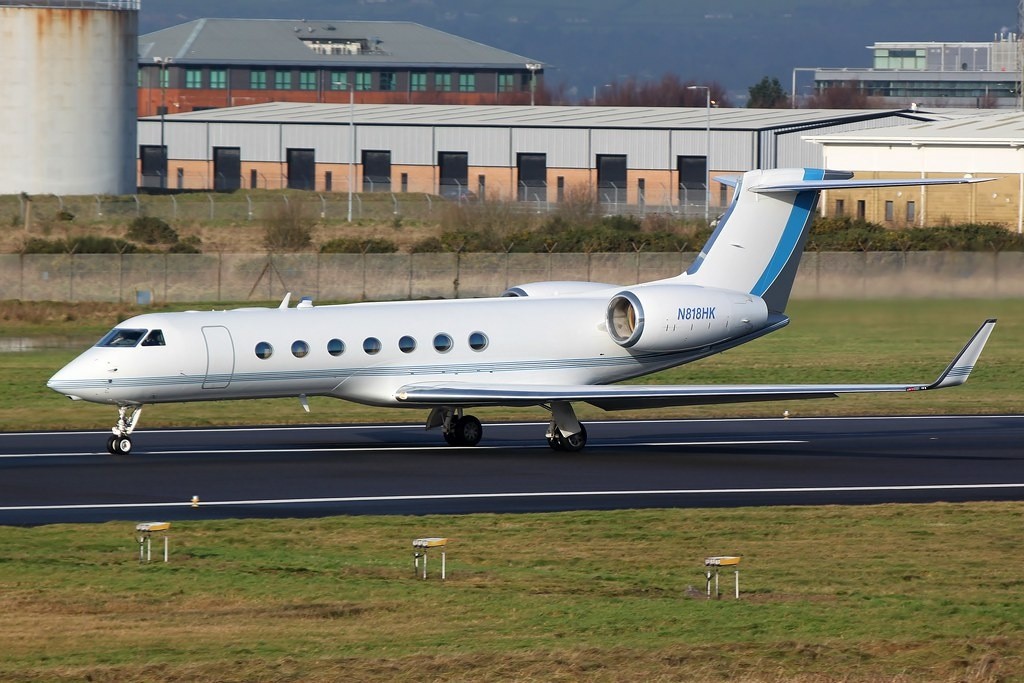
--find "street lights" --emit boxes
[332,81,354,223]
[524,63,542,107]
[152,55,174,189]
[687,85,711,220]
[592,83,614,104]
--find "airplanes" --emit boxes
[42,167,998,454]
